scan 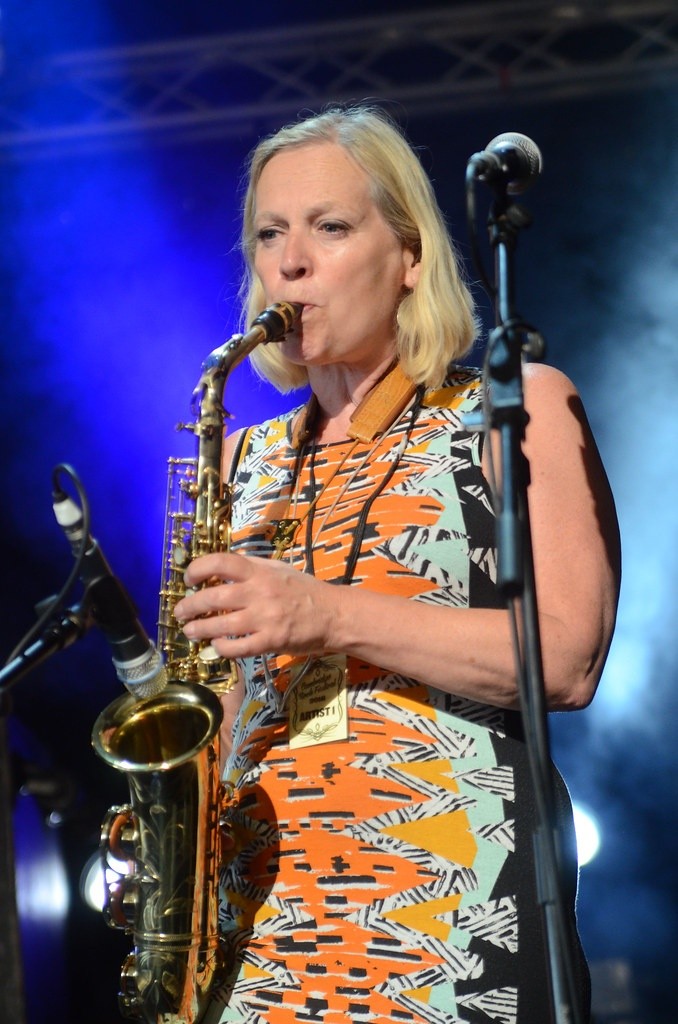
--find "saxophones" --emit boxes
[94,299,301,1023]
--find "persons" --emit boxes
[173,107,622,1023]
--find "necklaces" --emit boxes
[259,362,421,715]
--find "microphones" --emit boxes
[466,133,543,194]
[48,479,173,695]
